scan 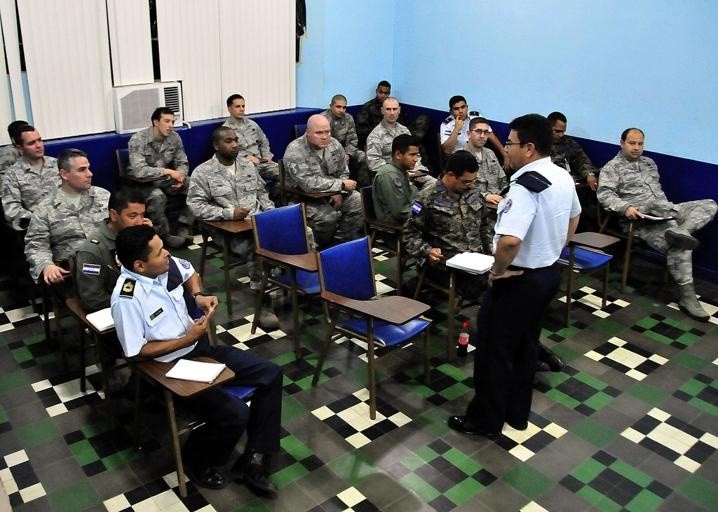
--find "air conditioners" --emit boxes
[113,81,183,135]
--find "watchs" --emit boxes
[192,291,209,299]
[488,265,504,276]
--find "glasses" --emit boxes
[473,129,490,134]
[505,140,521,146]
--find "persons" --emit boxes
[449,114,582,439]
[356,80,392,152]
[371,134,419,224]
[282,114,365,246]
[596,127,718,320]
[546,111,597,193]
[72,186,154,313]
[128,107,198,248]
[460,116,508,206]
[186,126,315,328]
[366,97,438,191]
[318,95,366,169]
[440,95,513,171]
[3,126,60,231]
[24,149,113,283]
[222,94,281,204]
[401,150,567,373]
[0,120,28,180]
[109,223,282,498]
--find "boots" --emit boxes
[664,226,700,249]
[252,293,280,331]
[161,231,186,249]
[678,284,710,322]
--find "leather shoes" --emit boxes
[241,463,279,499]
[447,415,504,436]
[184,464,225,489]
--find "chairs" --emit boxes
[438,131,454,170]
[121,273,262,498]
[250,203,332,361]
[593,183,668,292]
[66,255,161,428]
[574,180,603,228]
[311,235,433,420]
[294,123,308,140]
[274,158,343,207]
[359,186,416,296]
[36,261,86,375]
[116,149,172,204]
[183,176,254,314]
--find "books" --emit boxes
[165,358,226,385]
[445,250,494,275]
[86,306,115,332]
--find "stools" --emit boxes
[411,239,489,363]
[556,232,621,328]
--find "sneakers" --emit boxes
[537,346,567,371]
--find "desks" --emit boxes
[483,199,500,212]
